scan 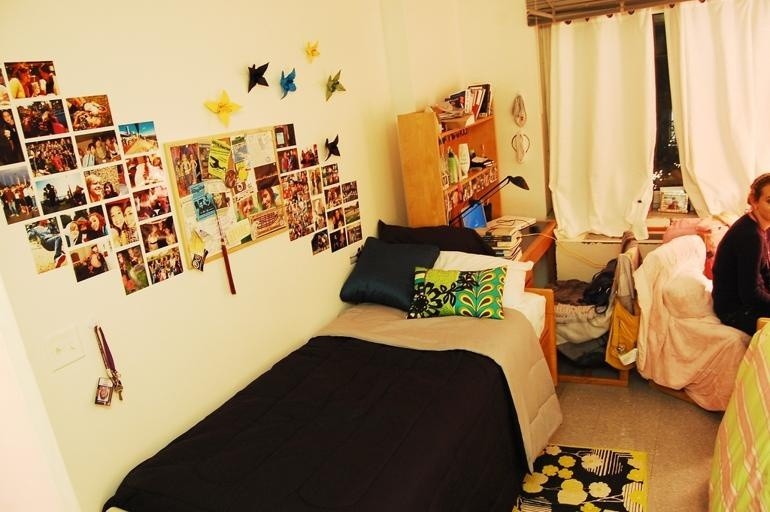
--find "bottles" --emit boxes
[458,143,470,177]
[441,119,447,132]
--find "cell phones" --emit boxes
[529,225,541,234]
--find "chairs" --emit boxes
[557,229,643,388]
[640,234,755,414]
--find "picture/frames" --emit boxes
[657,192,691,214]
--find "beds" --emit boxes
[704,318,770,512]
[96,278,559,512]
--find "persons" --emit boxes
[712,173,770,336]
[258,187,276,210]
[237,197,254,219]
[100,387,108,399]
[2,64,179,282]
[281,149,296,172]
[175,154,197,190]
[334,209,344,228]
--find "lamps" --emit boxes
[447,174,532,227]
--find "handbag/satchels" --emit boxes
[604,299,641,371]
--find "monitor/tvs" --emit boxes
[459,201,488,227]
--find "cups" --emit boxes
[439,123,443,133]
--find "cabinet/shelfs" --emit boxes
[398,97,557,294]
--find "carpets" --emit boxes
[512,439,650,511]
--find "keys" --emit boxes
[115,384,123,401]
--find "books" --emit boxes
[460,203,536,263]
[441,84,493,129]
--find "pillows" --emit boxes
[338,234,441,311]
[372,217,495,256]
[404,263,510,322]
[432,250,536,295]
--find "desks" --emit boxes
[564,201,726,244]
